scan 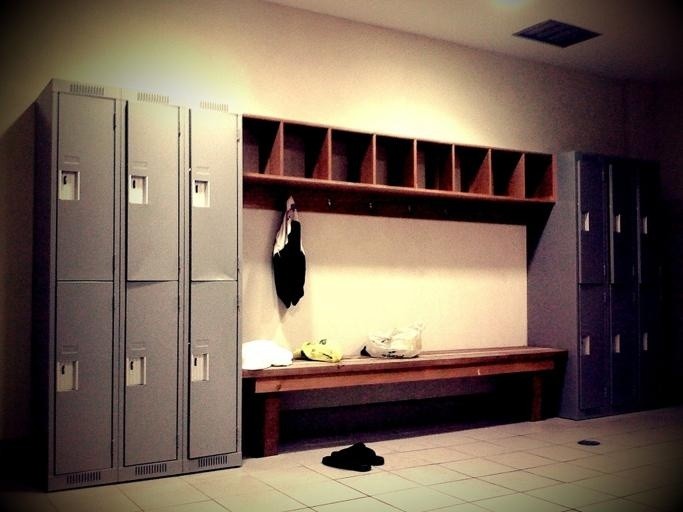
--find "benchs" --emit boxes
[240,342,567,461]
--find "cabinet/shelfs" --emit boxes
[643,290,672,414]
[241,109,558,216]
[188,93,244,286]
[114,284,183,488]
[638,153,664,288]
[526,287,612,422]
[28,77,115,280]
[527,144,611,286]
[28,282,112,495]
[185,281,244,473]
[613,288,643,416]
[610,154,637,288]
[112,84,183,282]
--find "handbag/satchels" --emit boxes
[366,321,423,358]
[293,339,342,362]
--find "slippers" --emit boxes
[322,456,370,472]
[331,442,384,465]
